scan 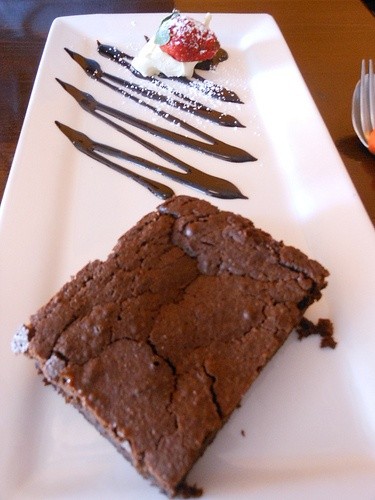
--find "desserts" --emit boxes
[131,10,222,79]
[13,195,333,500]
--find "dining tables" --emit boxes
[0,0,375,227]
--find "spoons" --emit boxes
[350,72,375,155]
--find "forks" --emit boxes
[358,55,374,159]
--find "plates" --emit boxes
[0,10,375,499]
